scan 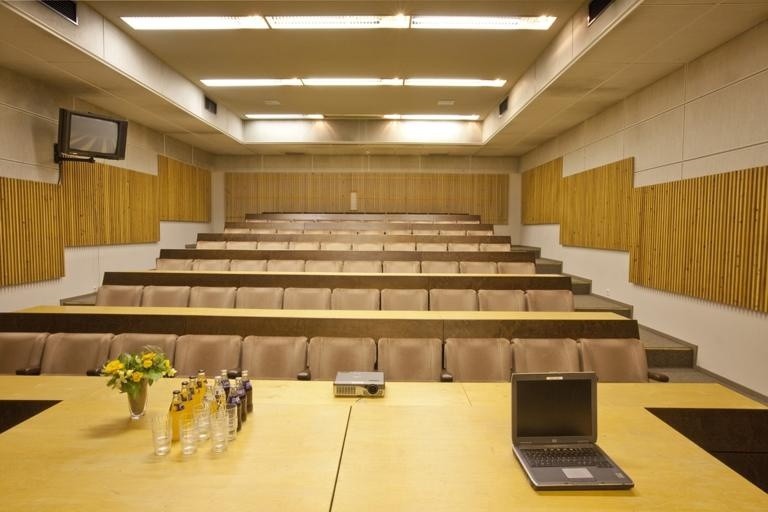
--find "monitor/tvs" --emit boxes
[58,109,127,161]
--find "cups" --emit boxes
[152,412,172,455]
[180,417,197,453]
[150,404,238,443]
[209,413,230,452]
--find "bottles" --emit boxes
[168,368,253,441]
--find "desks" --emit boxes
[330,381,768,512]
[0,370,353,512]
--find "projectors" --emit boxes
[333,371,386,398]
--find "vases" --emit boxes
[127,386,147,419]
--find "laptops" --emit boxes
[511,372,635,491]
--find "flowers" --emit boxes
[102,346,177,395]
